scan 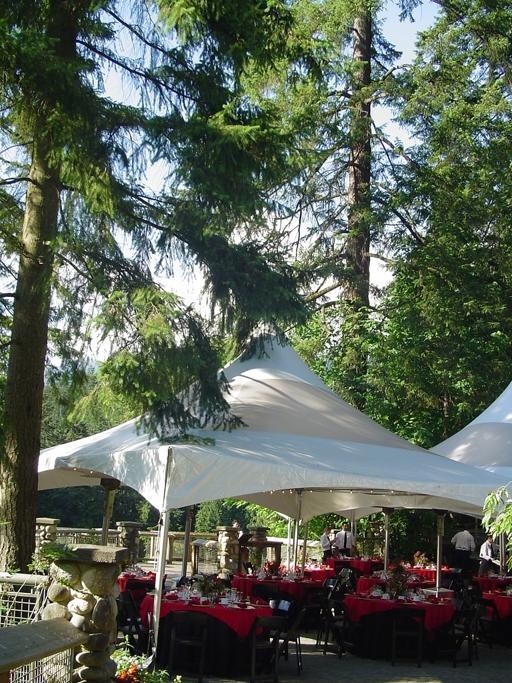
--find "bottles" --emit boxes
[236,592,247,608]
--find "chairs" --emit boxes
[115,562,512,682]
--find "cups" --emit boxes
[414,596,419,601]
[382,594,388,598]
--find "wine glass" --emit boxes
[184,581,192,601]
[290,573,296,583]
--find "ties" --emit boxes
[343,532,347,549]
[326,536,332,551]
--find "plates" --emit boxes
[228,604,256,610]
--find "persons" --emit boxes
[334,521,357,557]
[320,527,335,558]
[450,522,476,568]
[478,533,498,577]
